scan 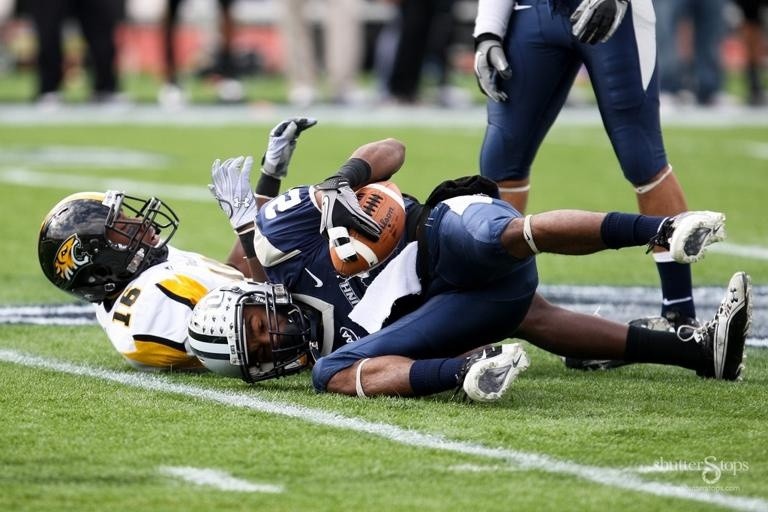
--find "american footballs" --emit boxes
[329,180,407,279]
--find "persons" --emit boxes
[470,2,695,330]
[35,115,748,385]
[186,140,726,401]
[2,1,767,106]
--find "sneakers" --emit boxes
[449,342,532,404]
[560,312,703,371]
[645,210,726,264]
[676,271,754,382]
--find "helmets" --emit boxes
[38,188,180,303]
[187,279,322,384]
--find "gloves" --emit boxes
[206,155,258,237]
[260,117,318,181]
[473,33,513,104]
[570,1,630,46]
[319,176,383,264]
